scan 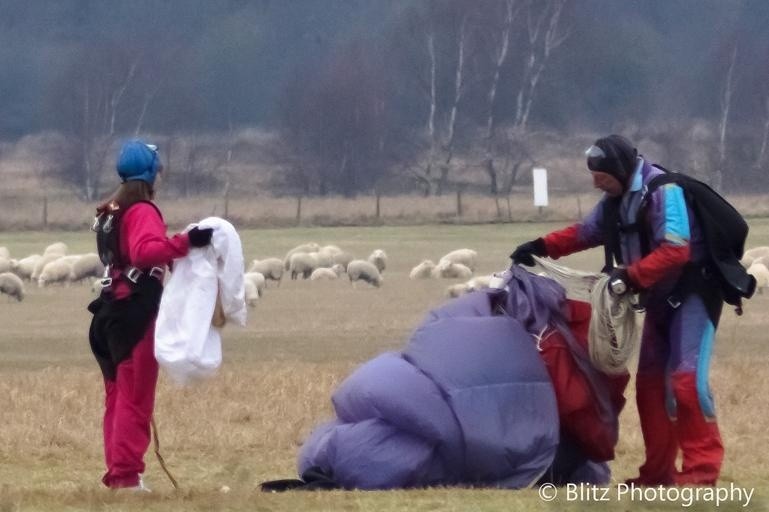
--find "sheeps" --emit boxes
[244,273,265,308]
[252,256,284,286]
[411,246,505,297]
[285,241,389,291]
[740,244,765,266]
[0,242,105,303]
[749,257,765,291]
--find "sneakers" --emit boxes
[117,473,152,494]
[625,477,675,486]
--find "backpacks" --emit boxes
[603,161,755,316]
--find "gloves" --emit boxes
[188,227,214,247]
[607,268,633,298]
[510,237,548,267]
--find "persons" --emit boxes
[83,139,215,494]
[509,132,724,490]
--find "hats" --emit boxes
[116,141,159,186]
[585,134,637,187]
